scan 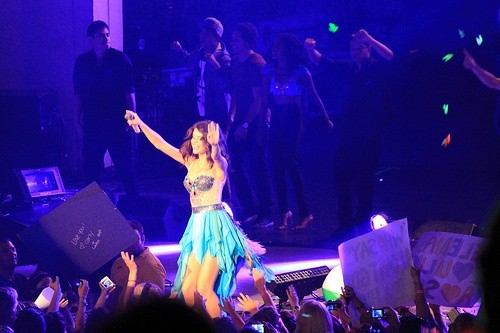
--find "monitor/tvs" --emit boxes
[13,166,66,202]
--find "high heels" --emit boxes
[295,214,314,229]
[279,210,293,230]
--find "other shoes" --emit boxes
[256,220,274,228]
[245,214,258,222]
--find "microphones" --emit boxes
[125,113,140,133]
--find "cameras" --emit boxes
[100,276,116,294]
[70,280,83,296]
[326,303,341,311]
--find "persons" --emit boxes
[461,49,500,91]
[204,22,274,228]
[73,20,135,196]
[124,109,267,318]
[0,213,500,333]
[258,33,334,231]
[303,29,393,227]
[170,16,231,203]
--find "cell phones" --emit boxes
[371,309,384,318]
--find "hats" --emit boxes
[201,17,224,39]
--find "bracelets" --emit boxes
[357,303,363,309]
[292,306,300,313]
[126,279,137,282]
[415,289,424,294]
[79,301,88,306]
[79,297,86,299]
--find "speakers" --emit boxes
[409,221,478,249]
